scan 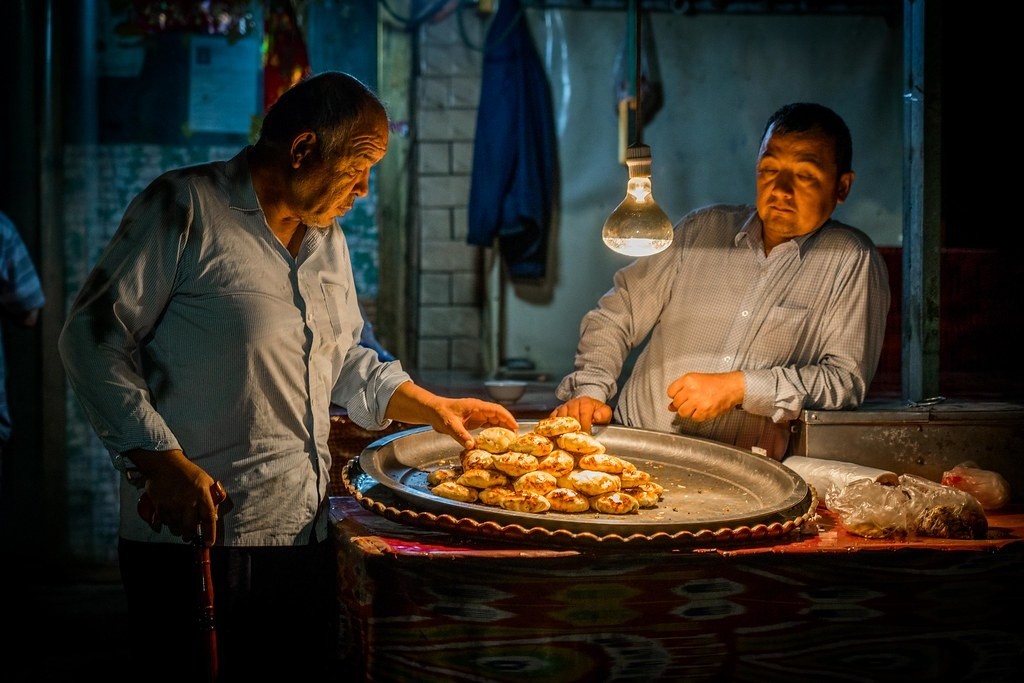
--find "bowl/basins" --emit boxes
[485,382,528,405]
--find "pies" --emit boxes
[426,416,664,514]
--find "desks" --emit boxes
[330,496,1024,683]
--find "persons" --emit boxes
[57,71,520,683]
[548,101,892,463]
[0,214,47,455]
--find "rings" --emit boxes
[206,513,217,522]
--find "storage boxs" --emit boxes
[786,395,1024,507]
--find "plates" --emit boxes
[360,419,807,533]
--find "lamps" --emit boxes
[602,12,673,257]
